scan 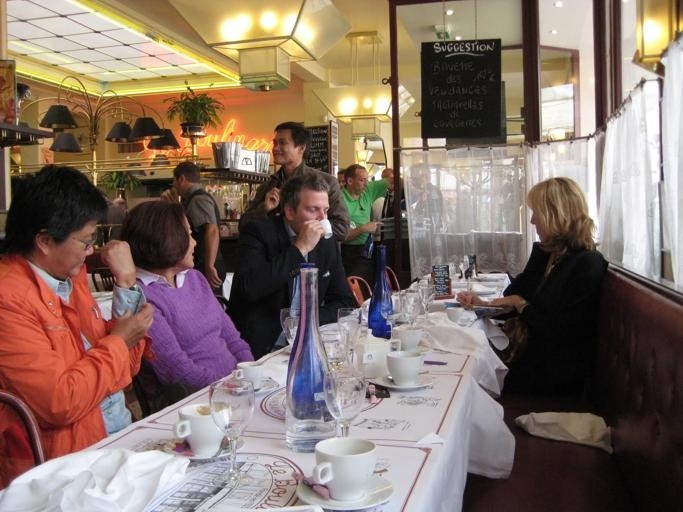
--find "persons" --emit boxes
[458,177,609,396]
[119,199,254,415]
[0,166,154,491]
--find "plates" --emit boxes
[214,379,279,393]
[162,440,241,462]
[473,289,498,295]
[295,472,396,510]
[372,376,436,390]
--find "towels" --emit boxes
[514,411,612,453]
[0,447,190,510]
[396,312,504,395]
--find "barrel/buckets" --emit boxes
[212,142,242,169]
[255,151,270,174]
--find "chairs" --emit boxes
[92,263,110,291]
[383,266,403,292]
[130,360,181,420]
[348,275,373,308]
[0,390,48,489]
[213,291,228,312]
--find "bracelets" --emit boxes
[516,300,528,313]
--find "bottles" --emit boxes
[284,263,339,453]
[368,245,393,339]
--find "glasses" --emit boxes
[73,238,97,250]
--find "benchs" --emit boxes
[469,262,680,510]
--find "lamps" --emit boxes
[15,74,180,191]
[170,1,352,91]
[631,2,679,84]
[310,30,417,140]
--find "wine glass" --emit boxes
[361,255,469,325]
[321,363,369,436]
[206,378,255,488]
[278,306,360,381]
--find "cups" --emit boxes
[311,435,379,501]
[235,360,263,392]
[391,325,429,350]
[385,351,424,386]
[175,401,232,464]
[318,219,333,241]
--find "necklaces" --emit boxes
[548,246,568,268]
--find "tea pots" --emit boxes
[353,326,401,380]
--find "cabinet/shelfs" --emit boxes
[174,169,272,240]
